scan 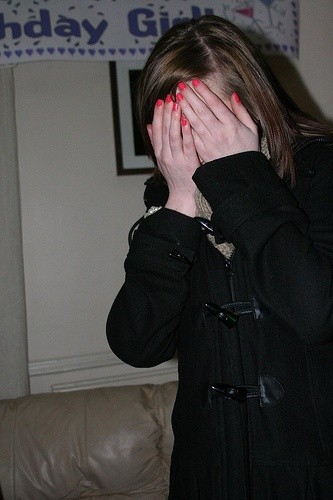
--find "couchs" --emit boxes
[0,380,179,499]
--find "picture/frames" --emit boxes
[108,57,163,177]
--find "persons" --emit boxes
[106,16,332,500]
